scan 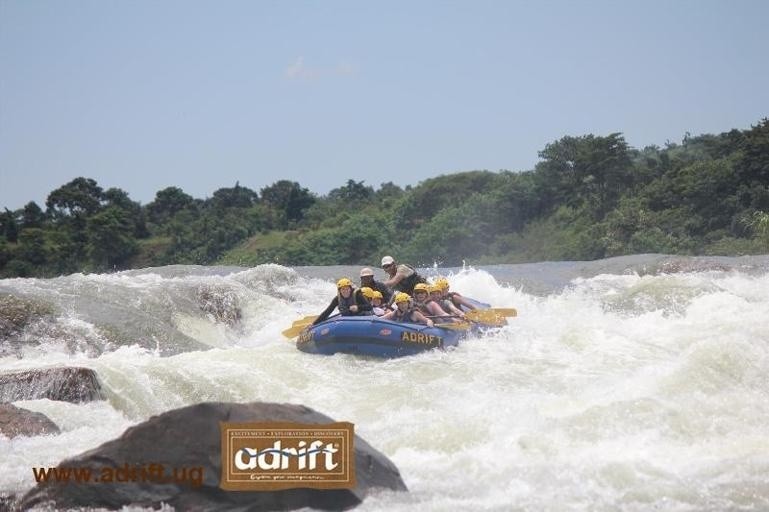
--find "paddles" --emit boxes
[424,308,517,333]
[283,312,342,339]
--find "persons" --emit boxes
[383,292,435,329]
[428,283,472,322]
[358,286,376,315]
[305,277,373,330]
[359,268,394,307]
[374,255,433,298]
[432,276,479,312]
[371,290,385,316]
[411,282,465,325]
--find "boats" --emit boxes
[296,296,494,359]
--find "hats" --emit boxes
[381,256,394,266]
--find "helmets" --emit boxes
[337,278,352,289]
[361,286,384,300]
[414,279,449,296]
[360,268,373,277]
[395,292,410,305]
